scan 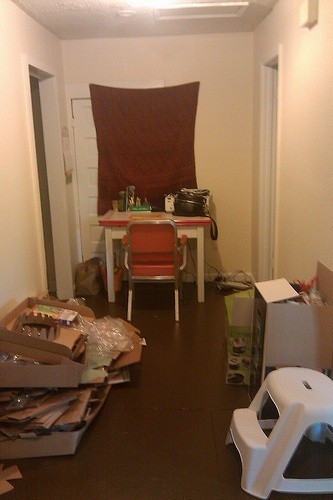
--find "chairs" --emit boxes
[121,220,188,323]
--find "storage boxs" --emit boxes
[222,259,333,420]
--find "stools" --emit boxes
[225,367,333,500]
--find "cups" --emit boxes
[112,200,119,211]
[119,191,125,211]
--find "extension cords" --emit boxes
[214,281,250,289]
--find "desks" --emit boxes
[97,209,213,303]
